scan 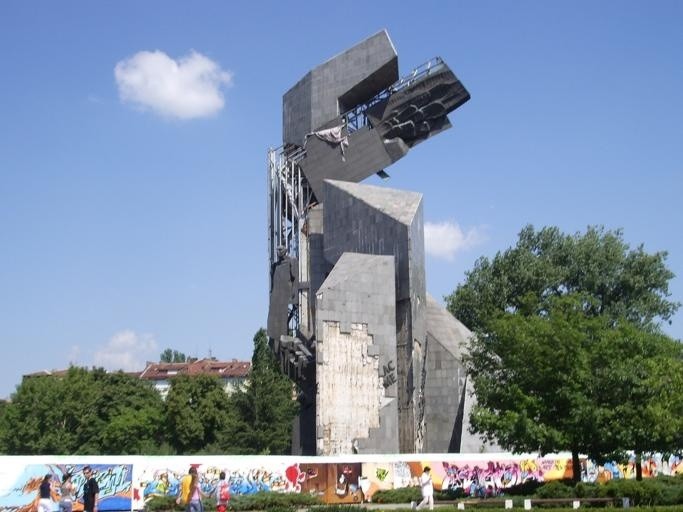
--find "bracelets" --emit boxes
[93,504,97,507]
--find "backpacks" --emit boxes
[219,482,230,500]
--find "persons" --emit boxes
[81,466,100,512]
[58,473,76,512]
[174,467,196,505]
[415,466,433,510]
[34,473,57,512]
[215,472,229,512]
[188,472,212,512]
